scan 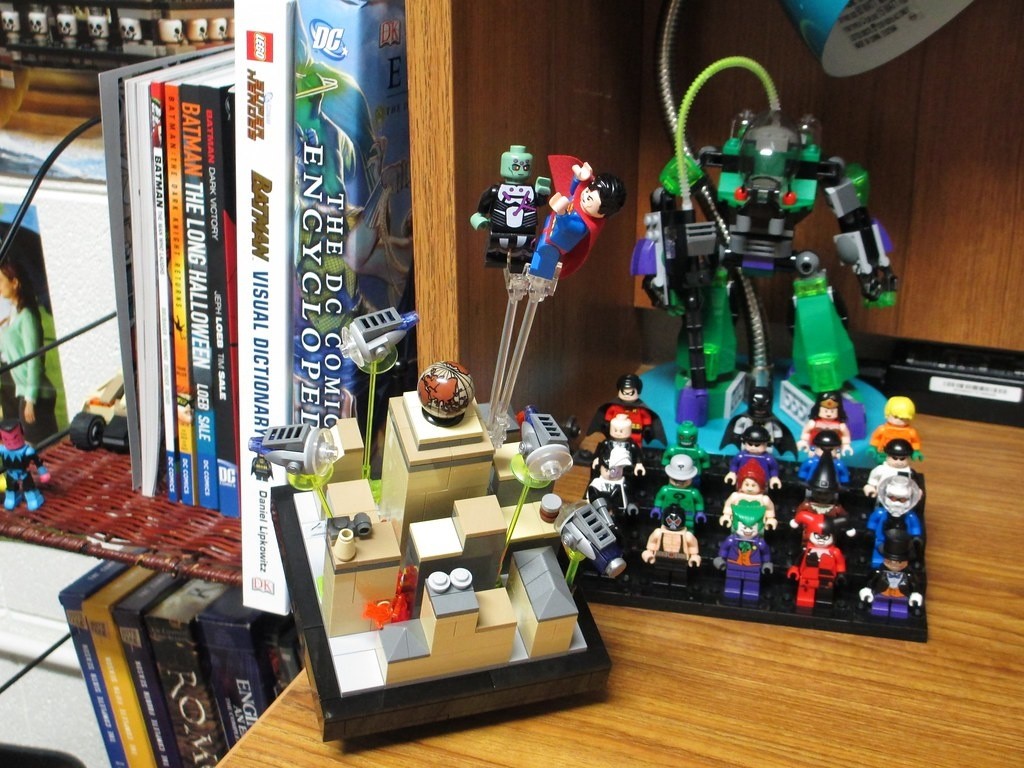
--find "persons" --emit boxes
[1,417,52,511]
[469,143,925,622]
[0,257,58,450]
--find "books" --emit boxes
[57,556,306,767]
[96,0,409,619]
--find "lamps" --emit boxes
[603,0,974,469]
